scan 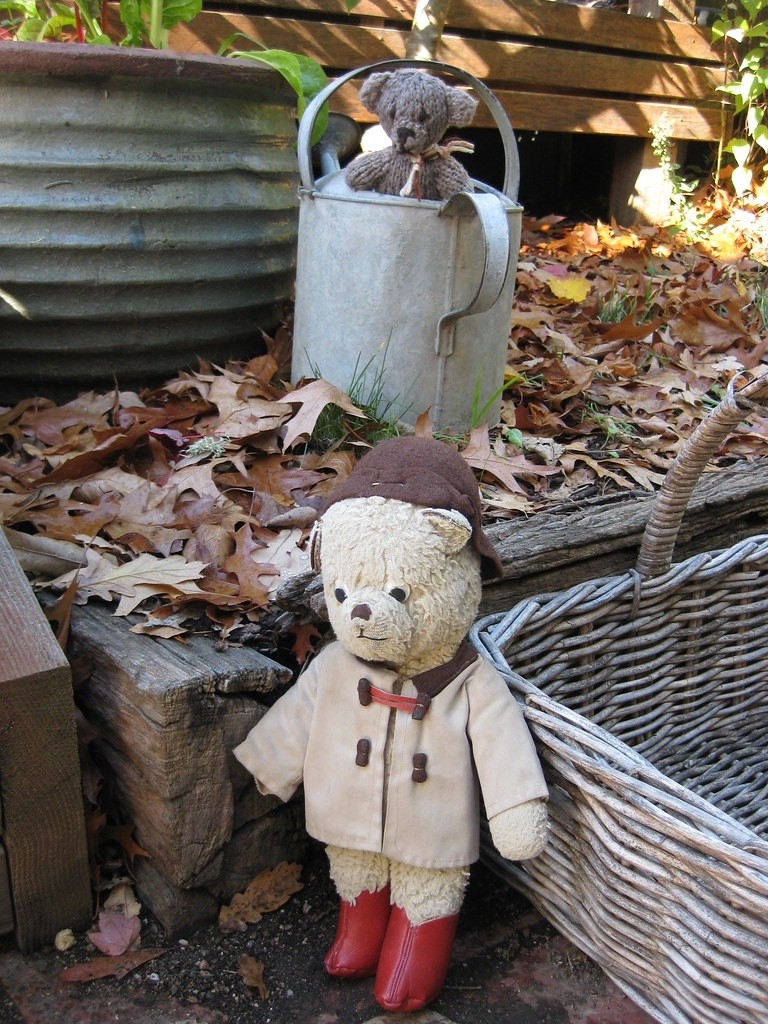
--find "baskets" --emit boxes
[467,369,768,1024]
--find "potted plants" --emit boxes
[0,0,336,416]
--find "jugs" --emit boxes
[297,60,527,445]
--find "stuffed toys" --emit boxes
[344,68,480,205]
[230,435,551,1010]
